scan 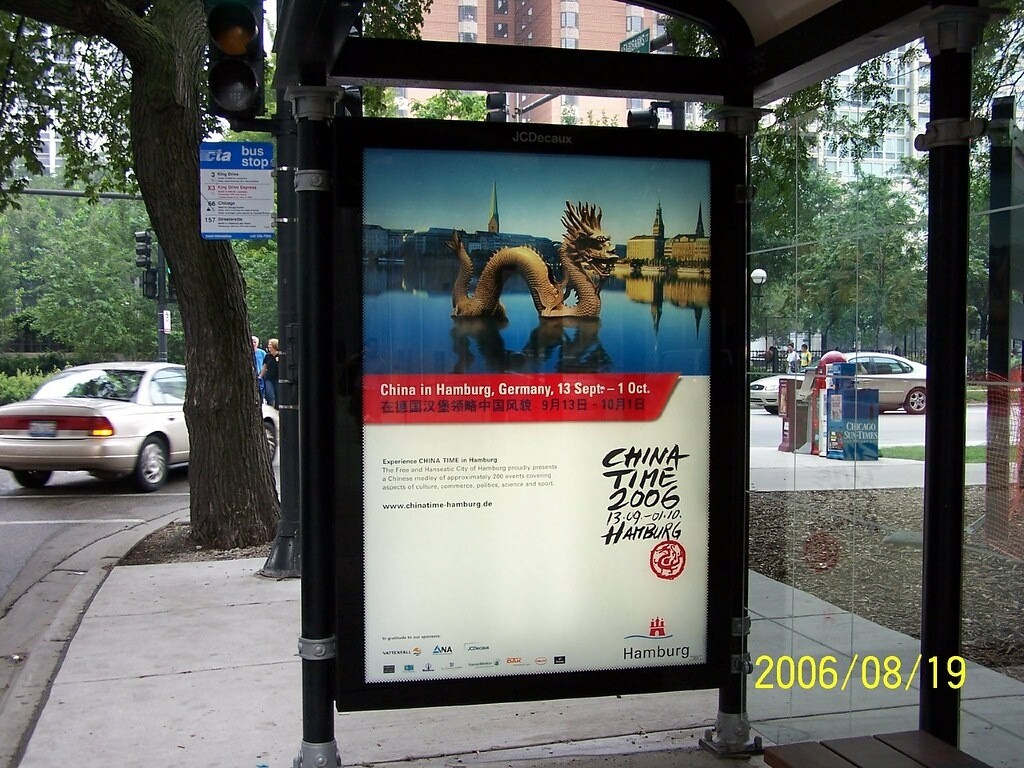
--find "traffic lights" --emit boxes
[206,0,268,123]
[142,269,158,299]
[135,231,152,268]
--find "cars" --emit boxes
[0,361,282,493]
[749,352,928,415]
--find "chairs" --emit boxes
[98,382,118,397]
[877,365,892,374]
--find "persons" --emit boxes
[787,343,800,374]
[259,338,279,409]
[252,335,266,399]
[801,344,812,368]
